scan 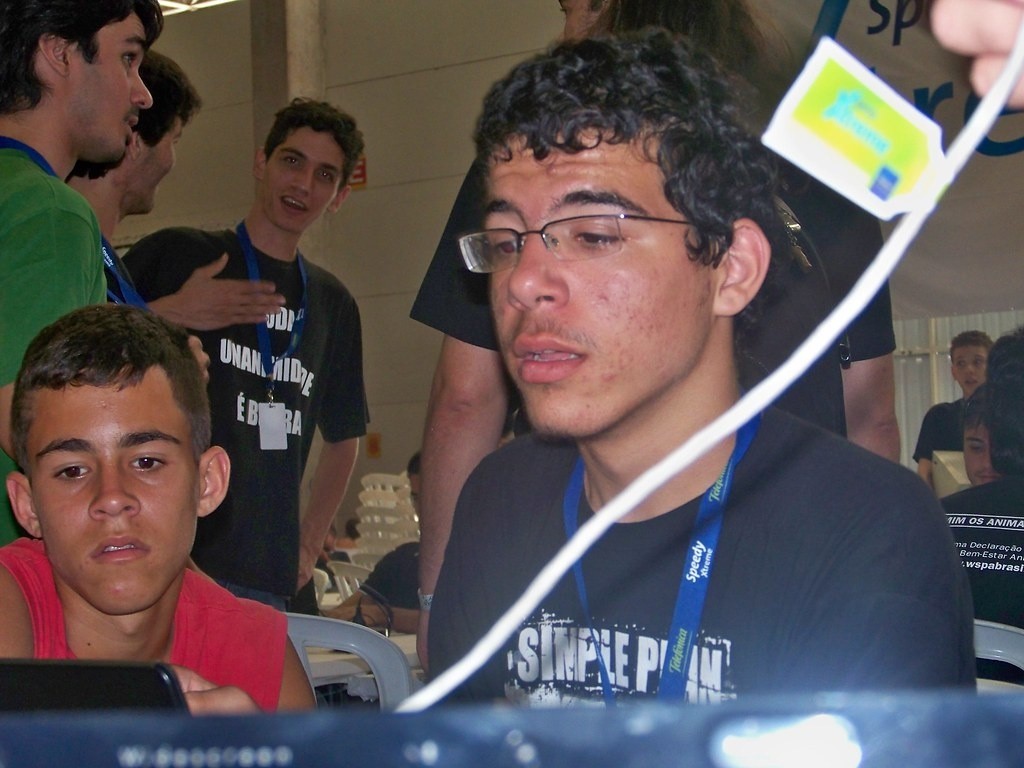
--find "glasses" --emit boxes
[455,212,728,274]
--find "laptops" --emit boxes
[0,660,192,717]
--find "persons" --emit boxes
[963,383,1002,486]
[0,0,978,714]
[939,329,1024,686]
[321,521,337,565]
[338,516,360,552]
[930,0,1024,113]
[323,449,423,633]
[914,331,994,481]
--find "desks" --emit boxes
[305,623,422,678]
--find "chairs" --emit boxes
[313,473,433,610]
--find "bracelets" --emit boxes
[417,589,433,610]
